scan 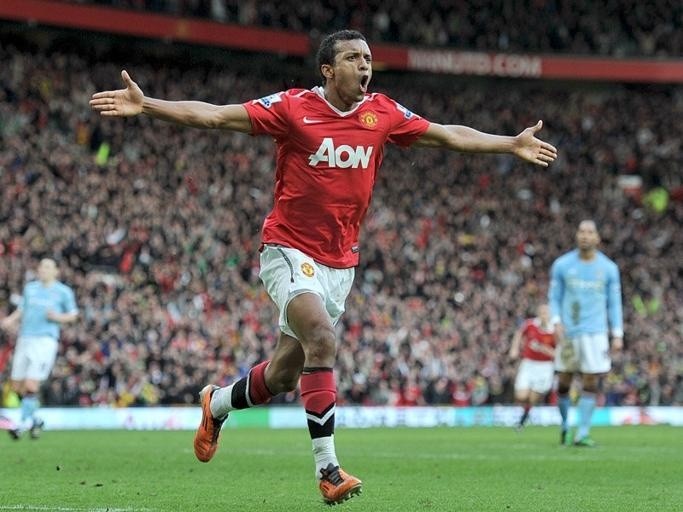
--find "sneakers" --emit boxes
[194,384,230,462]
[319,463,363,507]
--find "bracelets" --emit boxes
[55,312,62,324]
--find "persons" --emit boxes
[1,0,683,407]
[86,25,561,507]
[0,257,79,443]
[506,304,557,431]
[548,217,624,449]
